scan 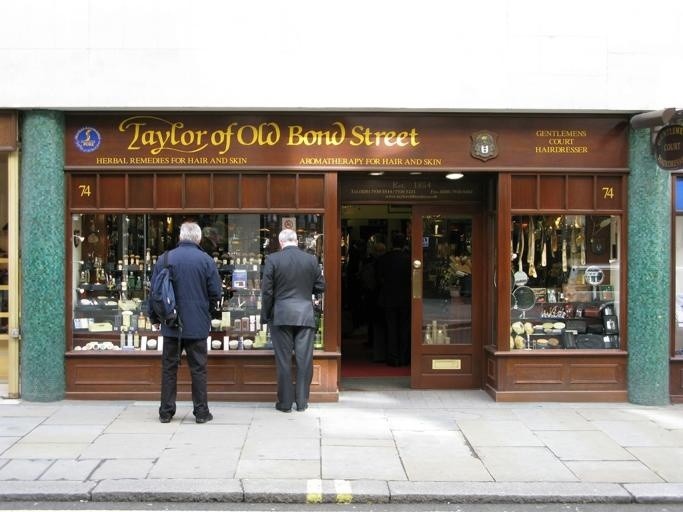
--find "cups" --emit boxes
[425,319,451,345]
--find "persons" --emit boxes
[144,221,222,423]
[256,227,326,412]
[371,231,409,368]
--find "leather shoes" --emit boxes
[196,414,213,423]
[298,404,308,411]
[276,402,291,413]
[159,415,170,423]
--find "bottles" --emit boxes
[250,290,256,311]
[257,293,261,309]
[127,330,133,346]
[134,330,139,348]
[119,329,126,346]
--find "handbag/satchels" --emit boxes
[151,250,180,327]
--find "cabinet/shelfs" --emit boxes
[73,263,324,351]
[510,300,620,349]
